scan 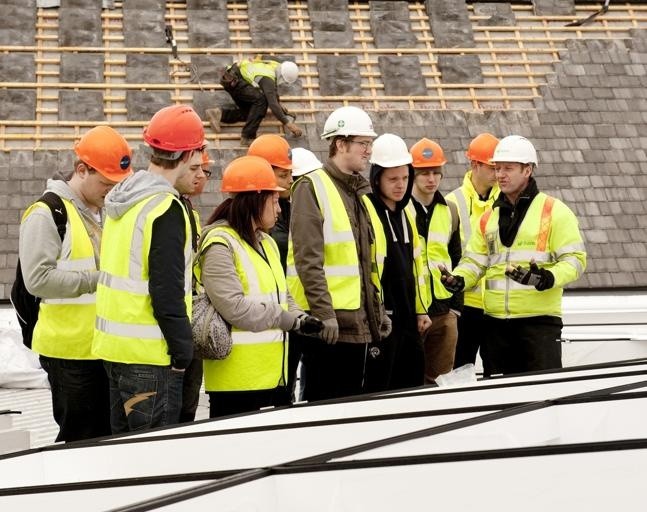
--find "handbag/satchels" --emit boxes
[189,291,234,361]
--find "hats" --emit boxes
[280,61,299,84]
[144,105,209,151]
[320,106,378,140]
[220,156,286,193]
[247,133,298,170]
[368,134,413,168]
[463,133,495,165]
[409,138,448,168]
[288,147,322,176]
[490,135,539,167]
[75,126,134,183]
[200,149,217,164]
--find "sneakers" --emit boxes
[238,136,255,147]
[206,108,222,134]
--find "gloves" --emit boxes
[505,263,554,295]
[299,315,326,334]
[286,122,303,137]
[318,317,340,345]
[437,266,466,295]
[376,314,393,338]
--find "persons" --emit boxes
[186,150,210,203]
[447,134,504,379]
[19,126,136,443]
[287,147,319,402]
[364,133,433,394]
[246,134,298,407]
[175,145,205,423]
[96,102,198,437]
[405,137,459,389]
[439,133,587,377]
[286,107,394,401]
[204,61,301,146]
[195,155,324,420]
